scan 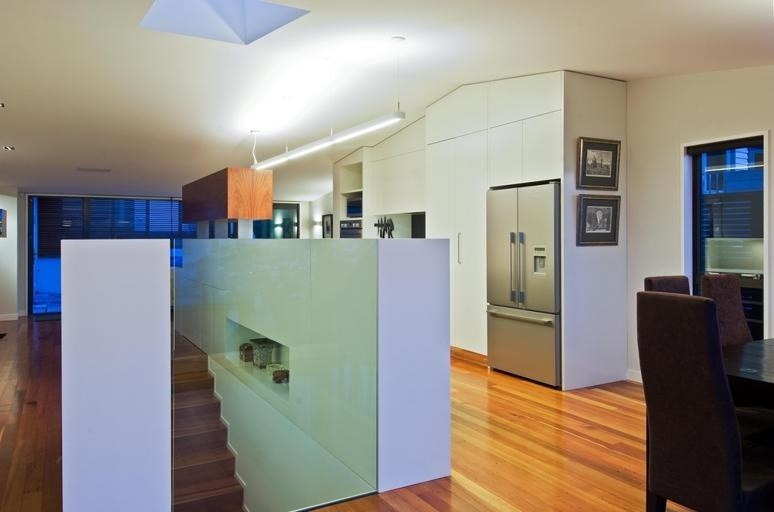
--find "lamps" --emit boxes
[247,99,407,172]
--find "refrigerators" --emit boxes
[484,184,562,391]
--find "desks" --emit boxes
[716,336,774,402]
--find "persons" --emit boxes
[592,209,607,231]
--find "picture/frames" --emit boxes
[321,213,334,239]
[575,135,622,191]
[575,194,622,247]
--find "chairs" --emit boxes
[643,275,691,295]
[635,290,773,511]
[699,274,752,347]
